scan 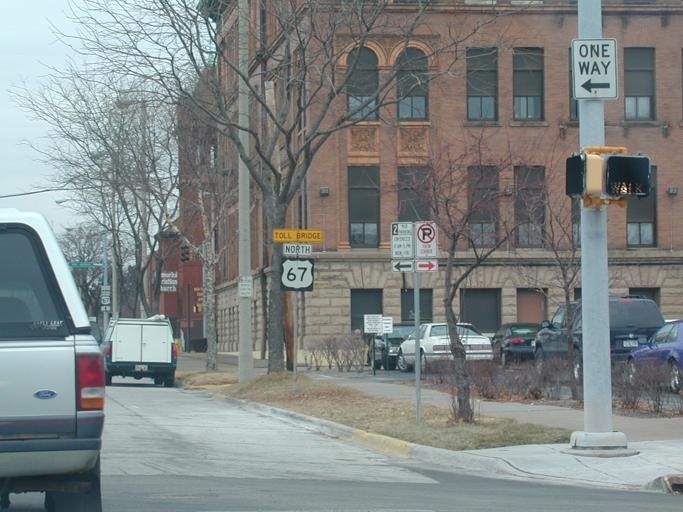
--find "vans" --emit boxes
[98,314,179,386]
[0,209,103,509]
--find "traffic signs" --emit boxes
[414,220,438,272]
[392,223,414,271]
[572,38,619,101]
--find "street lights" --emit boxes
[56,197,111,316]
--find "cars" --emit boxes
[396,321,495,369]
[491,323,544,366]
[367,321,416,370]
[626,319,682,393]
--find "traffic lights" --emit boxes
[607,156,650,196]
[565,153,585,198]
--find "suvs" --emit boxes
[530,296,666,388]
